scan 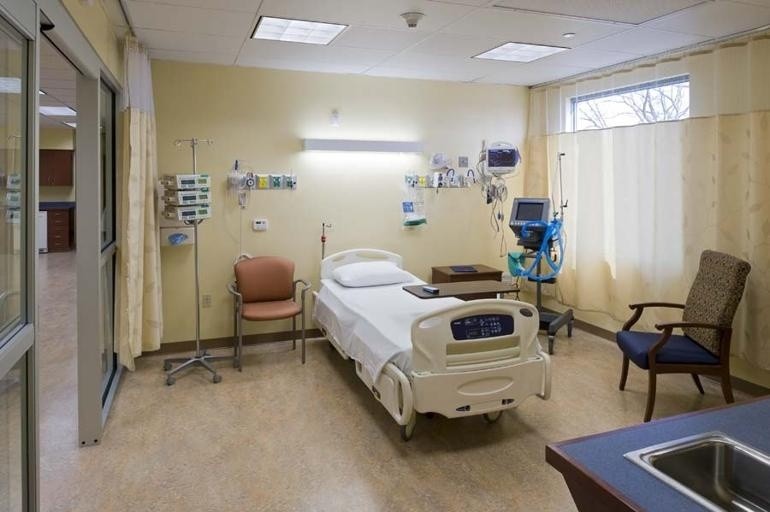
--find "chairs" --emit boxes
[226,254,310,374]
[616,250,751,425]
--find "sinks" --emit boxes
[623,430,770,512]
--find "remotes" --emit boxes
[422,286,439,293]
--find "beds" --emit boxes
[312,248,552,443]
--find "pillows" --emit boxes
[330,261,418,287]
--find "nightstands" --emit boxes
[431,266,503,301]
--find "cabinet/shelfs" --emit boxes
[40,150,73,186]
[46,207,74,252]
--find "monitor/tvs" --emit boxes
[486,147,519,174]
[509,197,551,238]
